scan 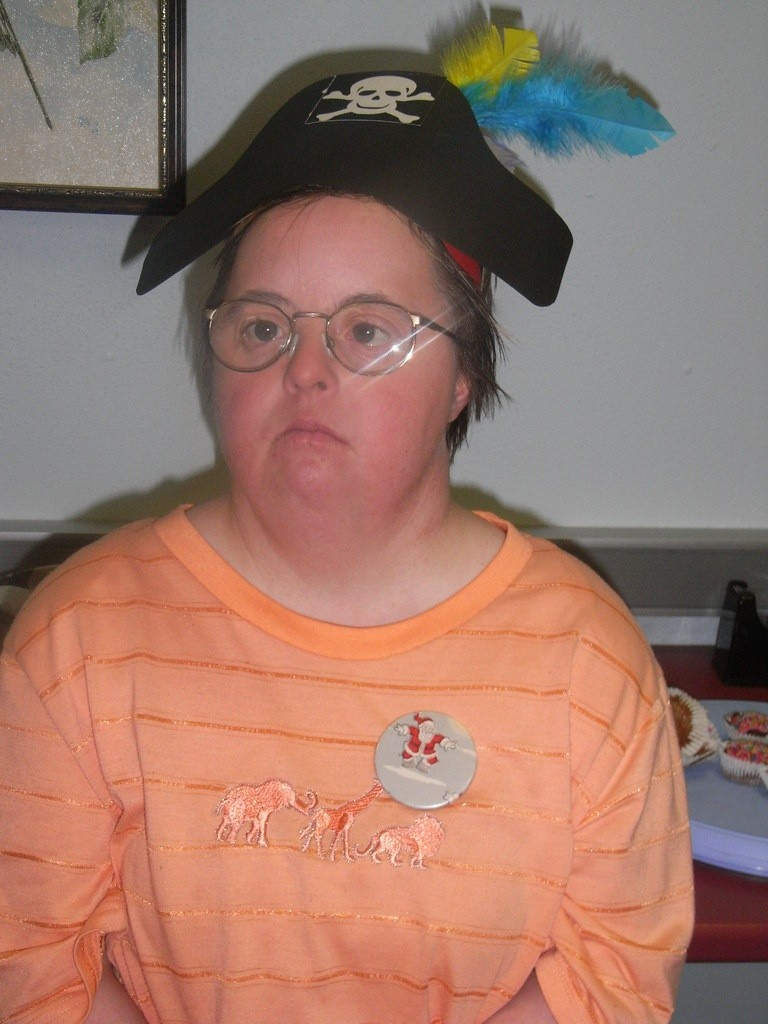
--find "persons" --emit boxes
[0,190,695,1024]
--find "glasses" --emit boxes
[206,300,458,377]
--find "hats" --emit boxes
[137,71,574,307]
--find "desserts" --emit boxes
[715,709,768,786]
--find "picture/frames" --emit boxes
[0,0,189,215]
